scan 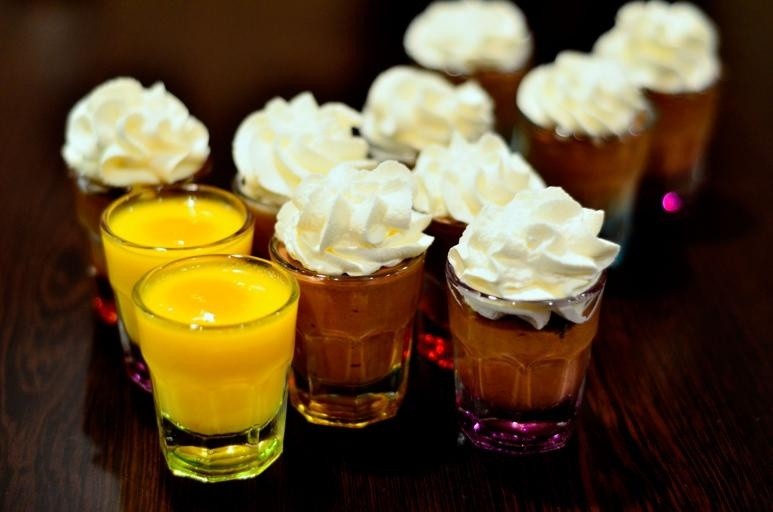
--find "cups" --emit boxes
[230,175,282,260]
[414,219,467,372]
[59,146,212,327]
[129,253,301,486]
[96,182,254,396]
[406,52,533,146]
[267,232,426,431]
[639,63,722,213]
[511,102,653,271]
[443,256,608,457]
